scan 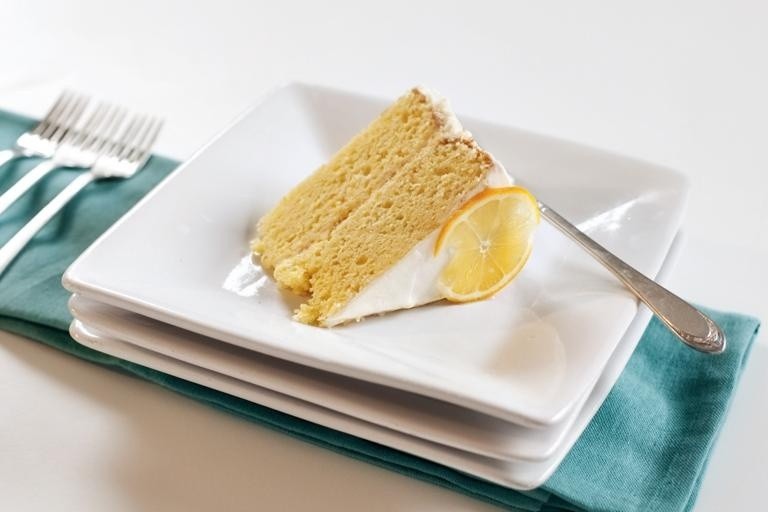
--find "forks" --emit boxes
[0,108,165,276]
[0,99,134,214]
[0,85,94,170]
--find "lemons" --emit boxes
[434,186,540,304]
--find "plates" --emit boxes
[68,218,688,493]
[60,74,698,433]
[61,292,613,464]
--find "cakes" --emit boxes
[254,91,540,326]
